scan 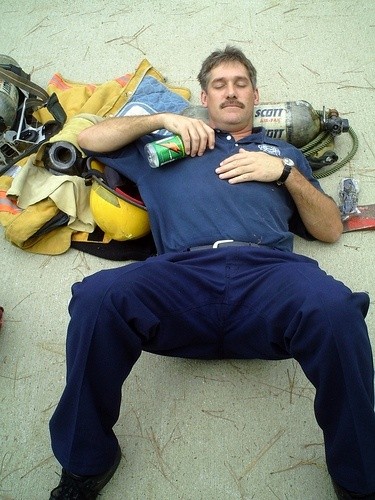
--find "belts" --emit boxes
[189,239,273,251]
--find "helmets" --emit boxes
[89,175,153,241]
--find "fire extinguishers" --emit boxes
[0,53,22,139]
[180,99,350,149]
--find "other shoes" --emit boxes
[332,479,375,500]
[49,445,121,500]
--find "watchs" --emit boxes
[276,157,295,185]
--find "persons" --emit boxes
[47,45,375,500]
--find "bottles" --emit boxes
[146,135,186,169]
